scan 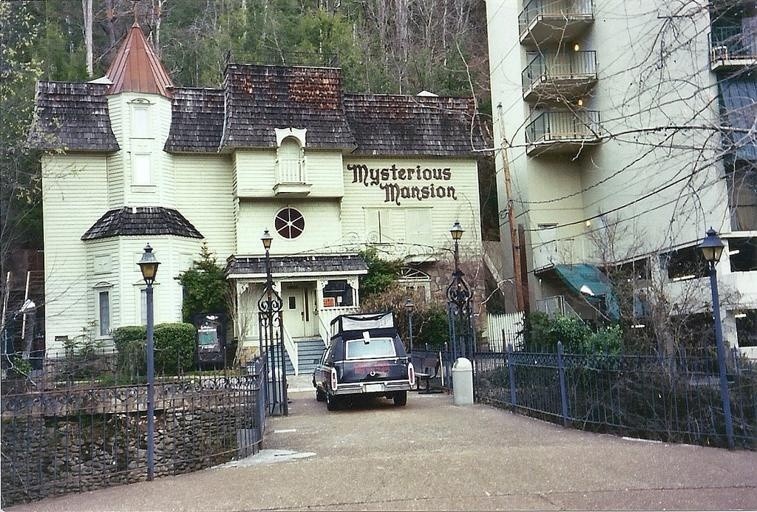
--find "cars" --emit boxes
[309,329,420,411]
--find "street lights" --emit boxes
[448,214,470,360]
[259,228,282,416]
[132,241,161,483]
[699,223,739,453]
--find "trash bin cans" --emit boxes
[451,357,474,406]
